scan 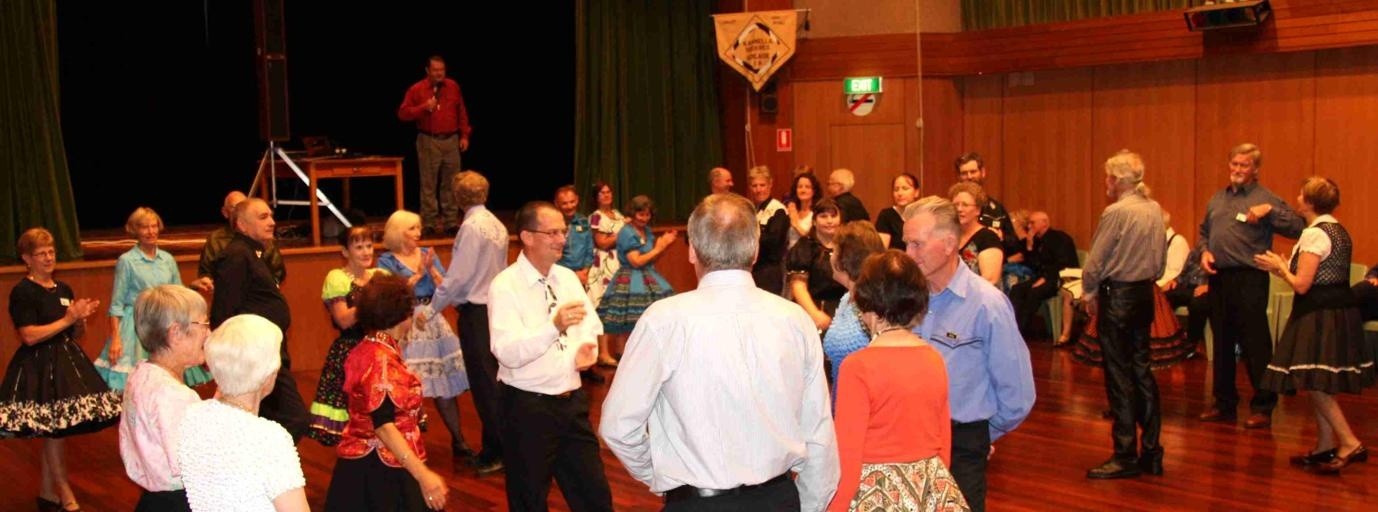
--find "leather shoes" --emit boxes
[1088,459,1139,478]
[1322,442,1368,470]
[1245,412,1271,427]
[1291,447,1337,465]
[1200,408,1236,421]
[1140,457,1163,475]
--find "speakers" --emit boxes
[761,77,778,112]
[255,1,291,141]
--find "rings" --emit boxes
[429,496,432,502]
[567,313,573,321]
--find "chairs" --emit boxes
[1043,244,1088,344]
[1205,262,1274,358]
[1279,260,1365,345]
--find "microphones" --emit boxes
[430,81,444,112]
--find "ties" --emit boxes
[539,278,568,349]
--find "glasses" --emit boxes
[192,317,211,327]
[527,228,568,239]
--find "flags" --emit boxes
[712,9,797,92]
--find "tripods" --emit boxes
[247,142,353,228]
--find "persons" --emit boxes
[1351,263,1378,324]
[376,210,478,466]
[1,228,123,512]
[197,191,287,289]
[416,171,509,476]
[1056,268,1085,346]
[93,208,213,396]
[903,195,1037,512]
[555,185,605,384]
[587,182,632,368]
[595,196,678,334]
[178,313,312,512]
[747,166,792,298]
[1082,148,1167,479]
[822,220,887,421]
[948,181,1007,295]
[785,197,843,384]
[1162,241,1209,360]
[1195,143,1306,429]
[1007,209,1030,264]
[598,193,841,512]
[307,275,450,512]
[684,168,734,247]
[119,285,213,511]
[1255,176,1369,475]
[875,173,920,252]
[782,172,824,302]
[955,152,1018,248]
[1155,208,1191,288]
[398,56,472,240]
[824,248,970,512]
[486,202,614,512]
[211,198,311,447]
[1009,211,1080,342]
[308,227,426,446]
[1070,282,1198,419]
[829,168,871,225]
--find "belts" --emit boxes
[504,384,583,400]
[421,130,458,140]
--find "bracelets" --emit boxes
[432,272,439,280]
[400,449,412,466]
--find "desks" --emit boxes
[261,158,407,246]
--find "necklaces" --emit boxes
[149,360,183,382]
[870,326,909,344]
[218,397,257,416]
[29,275,57,293]
